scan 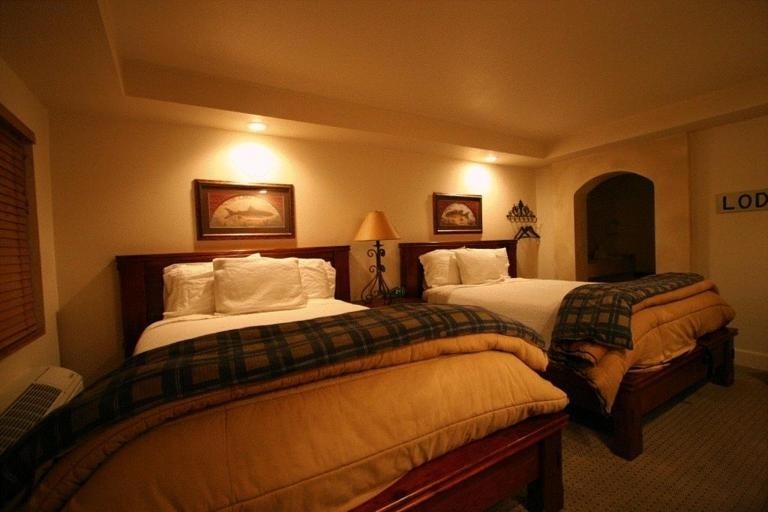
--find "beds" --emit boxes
[17,246,572,512]
[398,241,737,461]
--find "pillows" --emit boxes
[417,247,514,286]
[162,251,338,319]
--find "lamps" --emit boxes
[349,210,402,301]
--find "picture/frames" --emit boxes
[432,192,483,234]
[193,179,297,241]
